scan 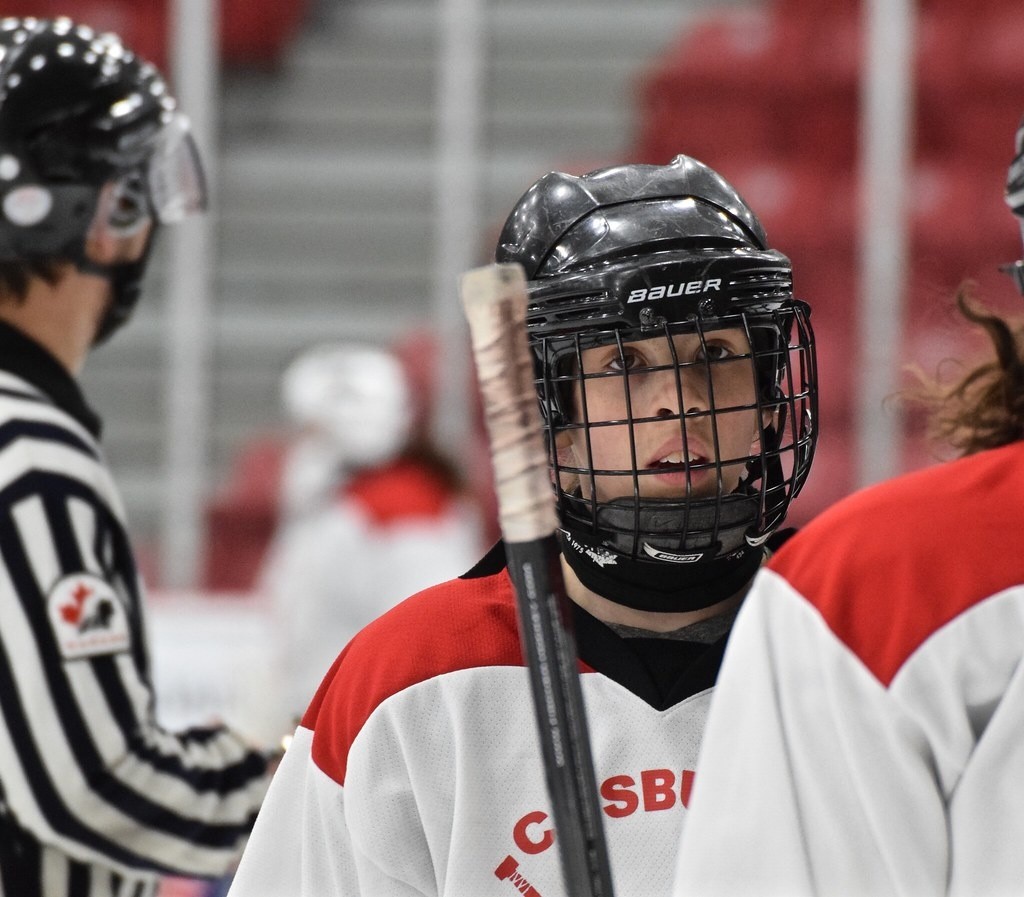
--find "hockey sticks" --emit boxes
[461,255,620,897]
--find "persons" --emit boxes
[679,112,1024,897]
[251,345,488,745]
[228,155,821,897]
[0,16,282,897]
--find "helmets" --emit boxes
[0,16,188,257]
[478,148,798,432]
[280,342,422,466]
